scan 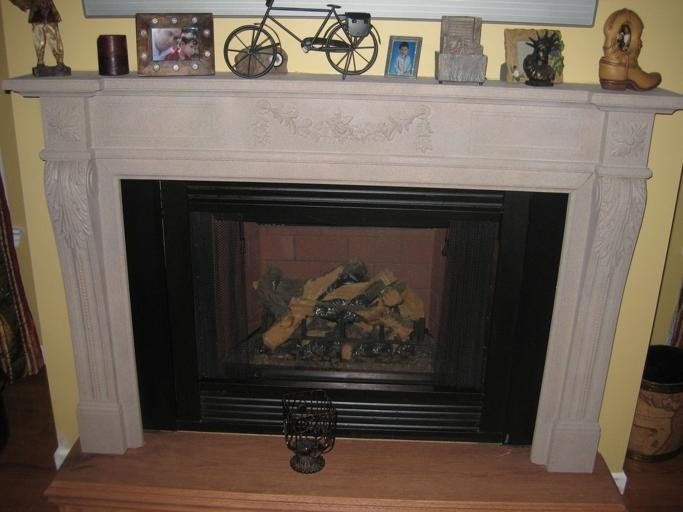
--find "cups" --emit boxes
[96,33,129,77]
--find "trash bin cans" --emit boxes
[626,345,683,463]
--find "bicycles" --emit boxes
[223,0,381,81]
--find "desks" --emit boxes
[40,425,626,512]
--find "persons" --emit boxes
[151,29,181,60]
[392,41,413,76]
[164,34,199,62]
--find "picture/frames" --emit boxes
[133,11,216,77]
[502,27,565,85]
[382,34,423,82]
